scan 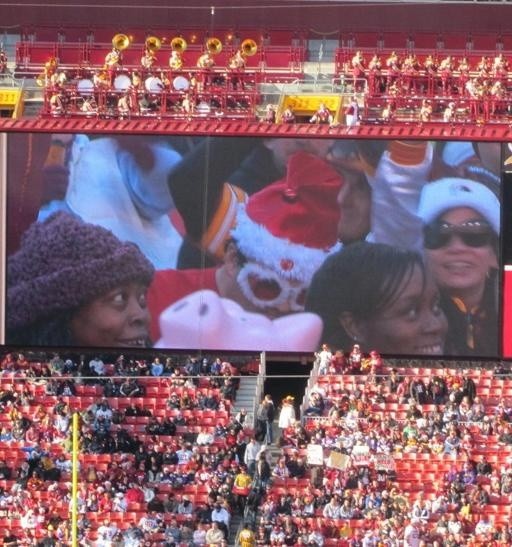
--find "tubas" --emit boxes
[169,36,187,70]
[197,38,221,70]
[141,37,161,67]
[106,34,129,65]
[230,39,258,70]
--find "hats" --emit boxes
[230,152,345,284]
[417,178,500,237]
[6,210,155,332]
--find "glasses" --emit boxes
[235,253,309,312]
[422,218,494,249]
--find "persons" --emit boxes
[42,47,244,122]
[0,352,512,547]
[344,49,511,123]
[0,53,8,73]
[264,103,333,123]
[5,133,502,353]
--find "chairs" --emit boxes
[1,362,512,547]
[0,26,512,120]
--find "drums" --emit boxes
[173,77,189,92]
[112,74,131,90]
[77,79,94,97]
[144,76,162,93]
